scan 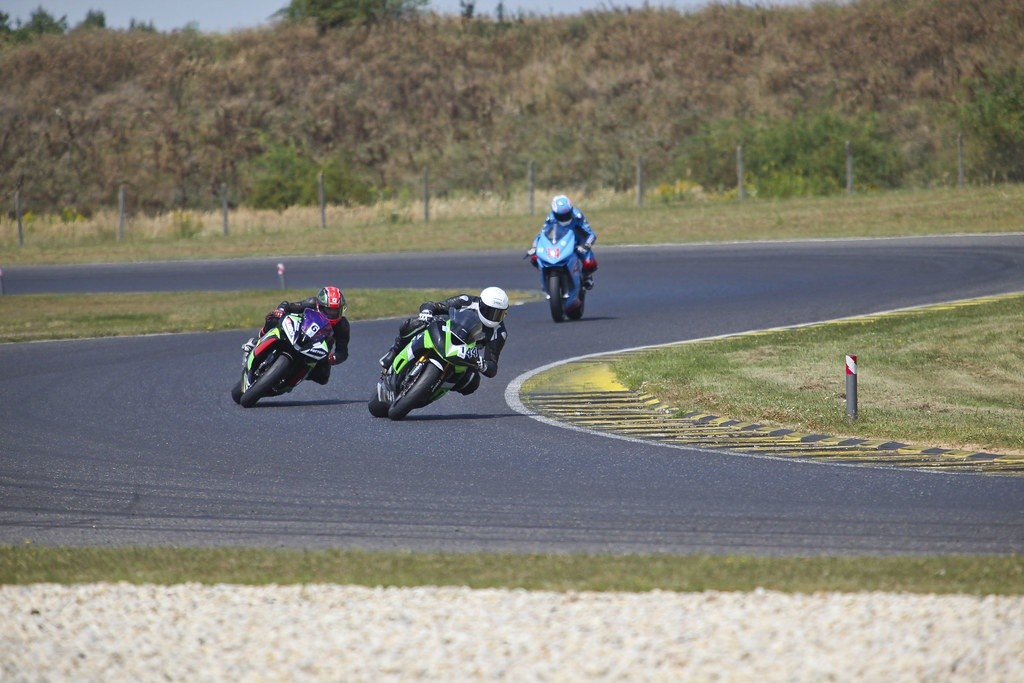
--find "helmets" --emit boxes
[477,286,509,327]
[316,286,346,326]
[551,195,573,226]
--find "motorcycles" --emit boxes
[368,309,482,422]
[523,225,589,322]
[230,307,336,408]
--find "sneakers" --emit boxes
[241,338,259,352]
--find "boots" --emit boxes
[379,336,413,369]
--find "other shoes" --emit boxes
[583,274,594,290]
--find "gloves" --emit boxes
[328,354,333,365]
[527,248,536,257]
[417,309,433,327]
[476,356,488,372]
[273,308,286,318]
[574,243,591,259]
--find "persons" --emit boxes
[378,285,509,397]
[240,285,351,386]
[527,193,598,293]
